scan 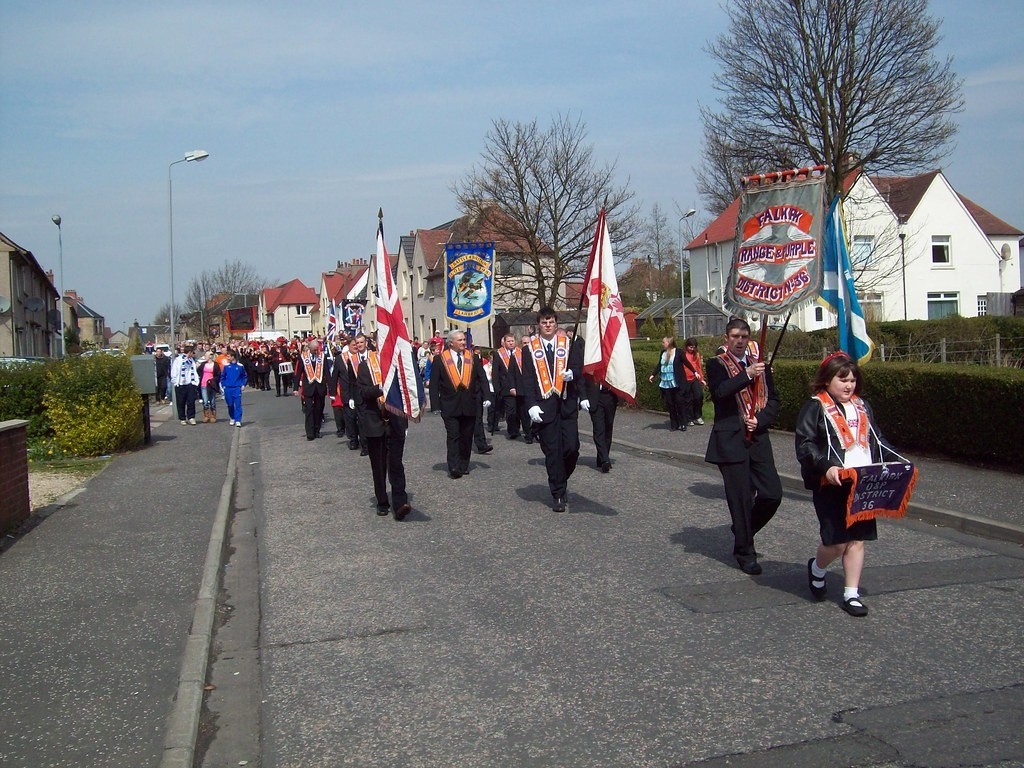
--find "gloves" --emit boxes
[527,406,544,424]
[580,399,590,411]
[483,400,492,408]
[348,399,355,409]
[562,368,573,381]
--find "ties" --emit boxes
[739,361,746,369]
[510,349,513,356]
[547,343,552,362]
[457,353,462,376]
[361,356,365,361]
[312,354,315,360]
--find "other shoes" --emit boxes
[451,468,469,478]
[671,418,704,430]
[843,598,867,616]
[378,502,388,516]
[479,424,540,454]
[602,462,609,472]
[396,504,411,519]
[808,557,827,596]
[304,430,368,456]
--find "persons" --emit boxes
[683,338,704,426]
[649,336,701,431]
[795,351,899,617]
[706,319,782,574]
[717,313,760,360]
[144,306,619,521]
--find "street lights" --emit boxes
[52,214,66,356]
[193,309,204,342]
[678,209,696,340]
[169,149,209,419]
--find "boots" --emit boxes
[210,410,216,423]
[203,409,210,423]
[246,379,299,398]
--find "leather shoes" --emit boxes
[735,560,762,575]
[553,498,566,511]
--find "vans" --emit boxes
[80,348,125,357]
[153,344,171,356]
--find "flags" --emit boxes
[582,208,636,403]
[324,300,336,378]
[815,195,874,366]
[375,222,428,423]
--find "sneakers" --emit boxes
[230,419,242,427]
[181,418,196,425]
